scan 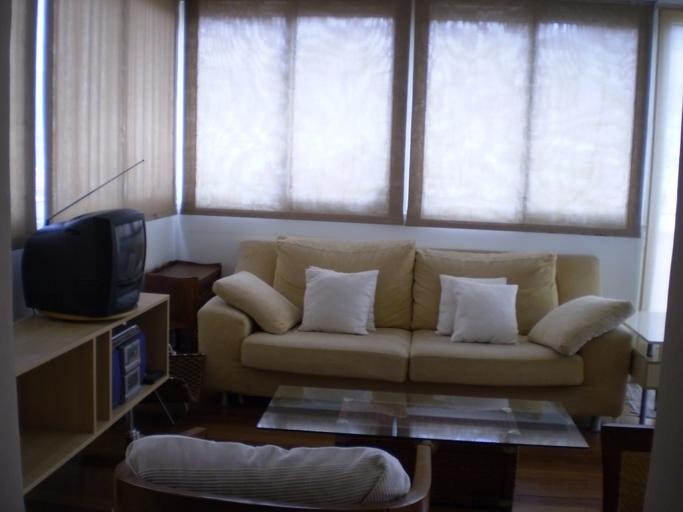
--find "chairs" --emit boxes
[113,428,431,512]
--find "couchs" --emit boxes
[198,236,634,432]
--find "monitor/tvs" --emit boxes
[21,209,146,317]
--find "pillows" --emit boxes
[307,266,379,333]
[125,433,411,505]
[529,294,634,356]
[449,278,519,344]
[297,267,374,335]
[273,236,415,328]
[215,271,303,334]
[435,274,506,336]
[413,246,559,335]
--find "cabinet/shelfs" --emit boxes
[144,258,222,327]
[13,293,170,496]
